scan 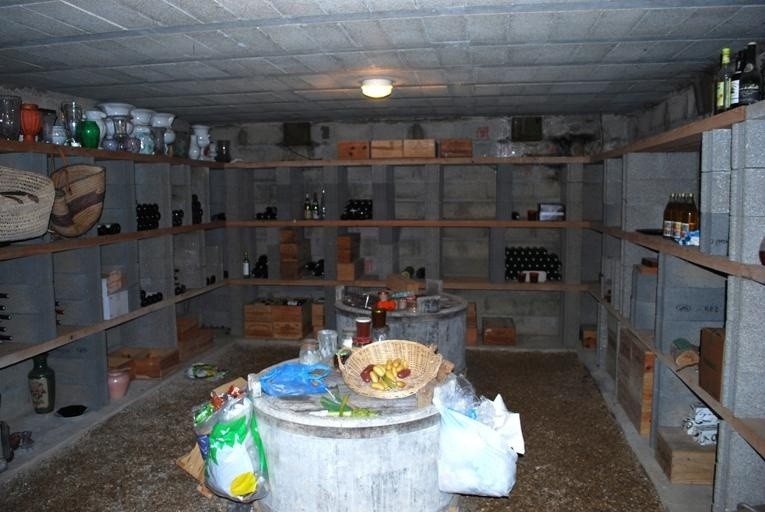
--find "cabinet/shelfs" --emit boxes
[575,100,765,512]
[225,156,590,354]
[0,139,236,486]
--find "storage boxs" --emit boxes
[244,297,517,344]
[277,227,363,281]
[580,258,727,486]
[102,272,215,379]
[336,139,473,158]
[537,203,566,222]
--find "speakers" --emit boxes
[283,122,311,144]
[512,116,543,142]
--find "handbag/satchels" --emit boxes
[49,144,107,238]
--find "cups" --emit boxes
[315,329,339,364]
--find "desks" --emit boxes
[335,279,468,375]
[247,359,454,512]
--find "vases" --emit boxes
[0,95,231,164]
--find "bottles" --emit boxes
[189,135,200,160]
[99,189,373,326]
[0,291,65,342]
[714,39,765,113]
[512,209,520,222]
[29,355,56,414]
[79,120,101,150]
[662,193,700,243]
[52,125,67,145]
[353,292,418,351]
[401,266,425,280]
[108,369,130,402]
[506,246,562,282]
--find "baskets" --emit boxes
[336,339,444,400]
[0,166,56,243]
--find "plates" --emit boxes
[55,405,92,417]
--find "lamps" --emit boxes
[359,79,394,98]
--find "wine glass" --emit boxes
[299,344,321,386]
